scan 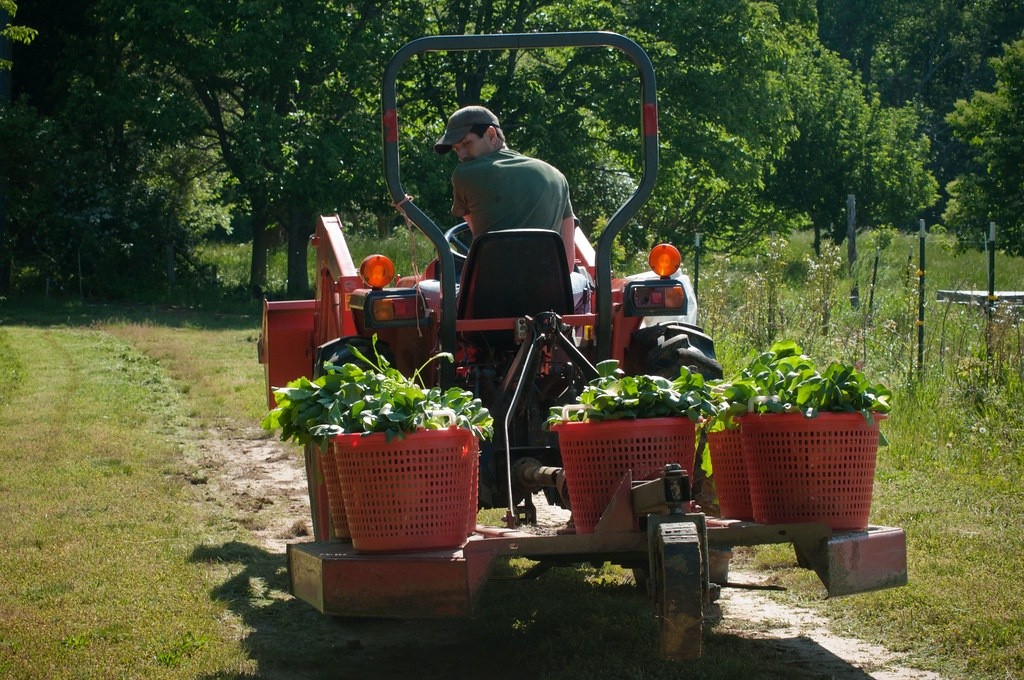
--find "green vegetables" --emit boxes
[543,336,894,476]
[261,333,495,442]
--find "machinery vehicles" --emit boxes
[256,29,910,668]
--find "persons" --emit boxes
[413,107,593,389]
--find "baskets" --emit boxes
[704,412,753,521]
[733,412,888,529]
[318,423,484,553]
[550,404,704,535]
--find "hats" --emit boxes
[433,106,499,155]
